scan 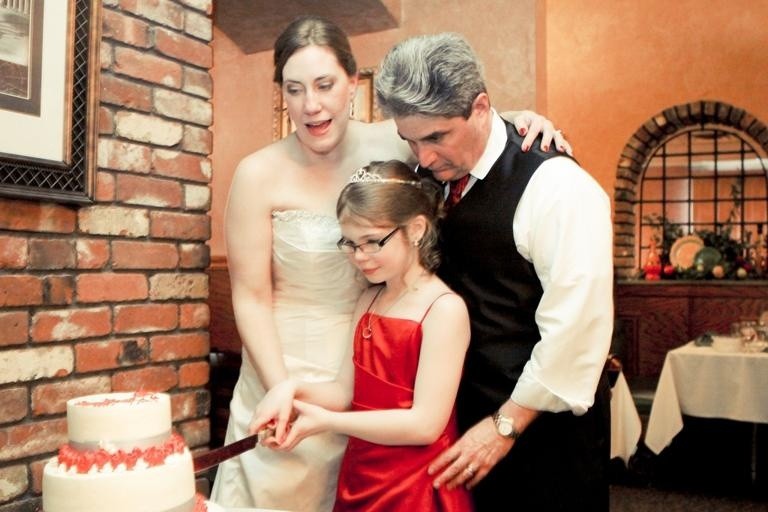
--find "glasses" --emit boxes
[336,224,402,256]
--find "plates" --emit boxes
[671,236,703,269]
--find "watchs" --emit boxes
[492,410,520,441]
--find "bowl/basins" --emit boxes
[731,321,768,357]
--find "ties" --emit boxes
[441,172,471,217]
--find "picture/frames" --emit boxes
[0,1,103,207]
[270,67,385,147]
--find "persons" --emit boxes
[207,15,574,511]
[247,158,474,512]
[370,31,613,512]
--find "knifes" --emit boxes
[193,412,295,473]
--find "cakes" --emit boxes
[42,391,207,512]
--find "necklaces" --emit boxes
[360,279,425,339]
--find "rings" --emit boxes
[554,129,563,137]
